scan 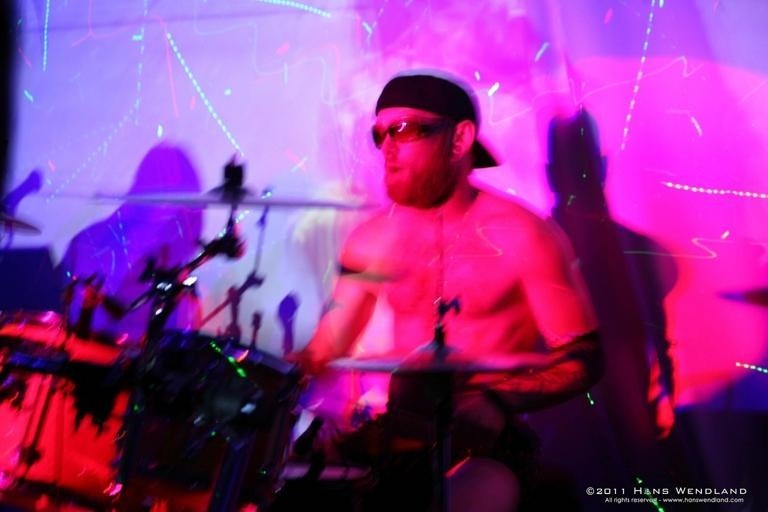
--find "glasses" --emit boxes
[371,120,452,149]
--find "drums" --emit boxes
[113,327,305,512]
[2,310,142,510]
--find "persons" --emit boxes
[281,72,597,512]
[47,141,206,356]
[542,108,675,442]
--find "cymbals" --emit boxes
[326,353,570,372]
[0,214,41,234]
[98,190,371,208]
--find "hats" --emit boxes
[374,75,497,169]
